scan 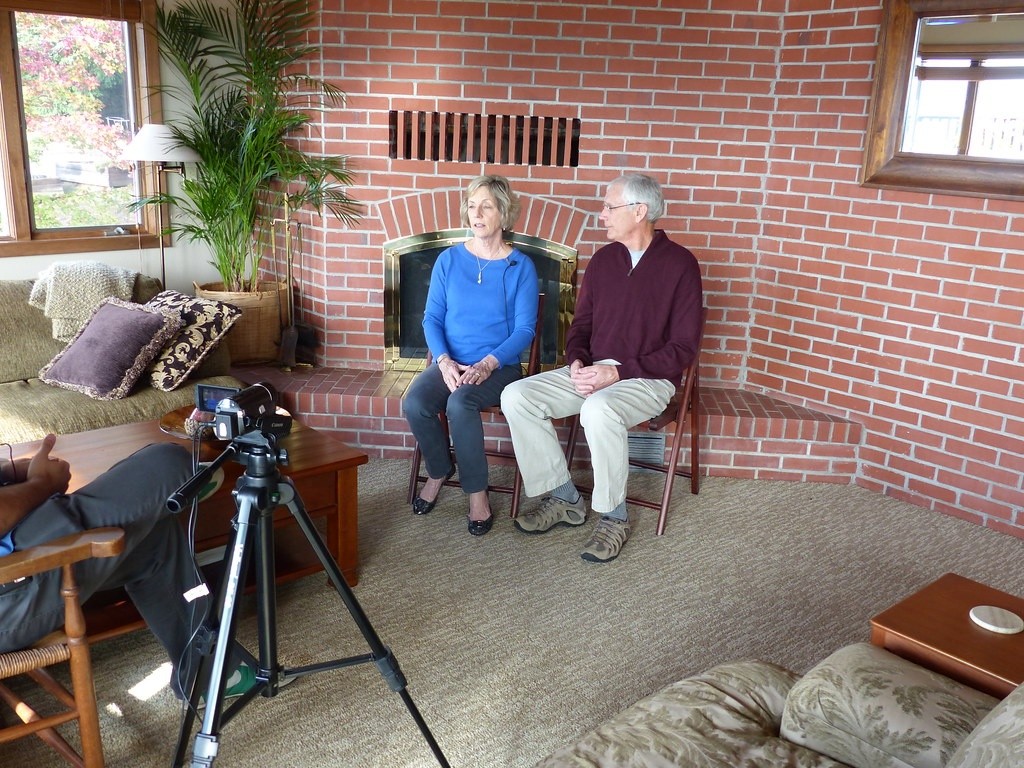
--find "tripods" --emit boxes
[166,432,451,768]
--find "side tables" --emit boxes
[869,572,1024,702]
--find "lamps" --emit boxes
[116,124,205,292]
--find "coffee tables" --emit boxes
[0,418,368,644]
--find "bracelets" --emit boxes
[437,355,449,364]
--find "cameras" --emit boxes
[194,382,278,440]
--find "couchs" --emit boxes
[527,639,1024,768]
[0,274,250,446]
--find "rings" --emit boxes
[476,373,481,377]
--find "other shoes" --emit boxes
[413,464,457,515]
[467,489,493,535]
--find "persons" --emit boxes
[207,392,217,408]
[499,175,703,563]
[0,434,258,710]
[404,173,539,533]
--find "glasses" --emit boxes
[603,201,640,211]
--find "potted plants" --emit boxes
[124,0,365,368]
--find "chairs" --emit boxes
[405,293,546,519]
[563,306,709,536]
[0,526,127,768]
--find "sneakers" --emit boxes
[195,463,225,505]
[581,508,632,562]
[178,659,297,711]
[513,495,588,535]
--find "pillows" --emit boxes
[37,296,183,403]
[142,289,243,392]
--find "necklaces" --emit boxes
[473,244,505,285]
